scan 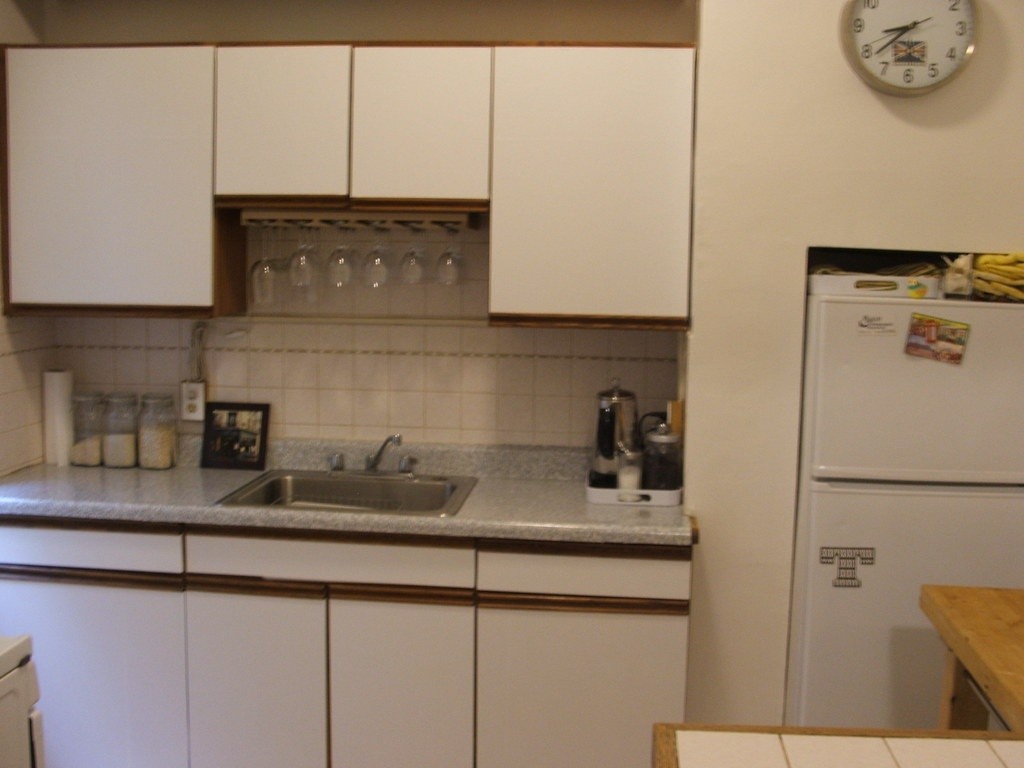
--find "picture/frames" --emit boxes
[199,401,270,471]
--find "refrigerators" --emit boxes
[784,295,1024,732]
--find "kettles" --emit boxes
[589,376,636,488]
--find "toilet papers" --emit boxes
[42,366,75,465]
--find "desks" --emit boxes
[917,584,1024,735]
[652,722,1024,768]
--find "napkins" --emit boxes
[675,730,789,768]
[781,733,899,768]
[884,737,1005,768]
[988,740,1024,768]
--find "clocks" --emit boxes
[838,0,979,98]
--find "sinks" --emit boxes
[211,466,480,520]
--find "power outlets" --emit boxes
[179,382,207,420]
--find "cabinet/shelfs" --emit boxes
[0,43,247,318]
[0,434,694,768]
[211,43,492,202]
[485,40,699,327]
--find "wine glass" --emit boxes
[241,210,470,308]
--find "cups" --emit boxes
[645,422,679,489]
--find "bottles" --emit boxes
[138,393,175,469]
[614,449,644,491]
[70,394,103,466]
[101,393,139,469]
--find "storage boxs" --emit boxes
[585,470,683,507]
[808,272,940,298]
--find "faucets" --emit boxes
[364,431,403,472]
